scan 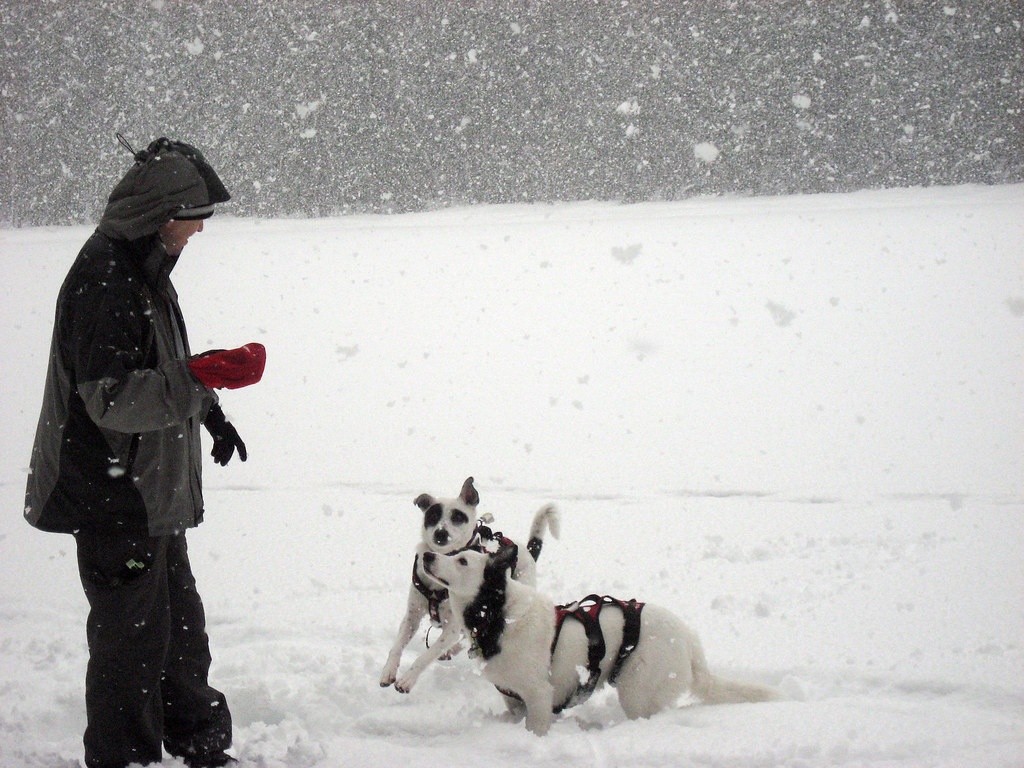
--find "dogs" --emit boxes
[379,476,780,740]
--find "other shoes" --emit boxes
[184,754,238,768]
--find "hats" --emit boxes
[173,202,214,221]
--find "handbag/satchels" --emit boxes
[84,474,156,587]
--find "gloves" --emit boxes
[203,404,247,467]
[189,343,265,391]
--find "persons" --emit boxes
[23,132,267,768]
